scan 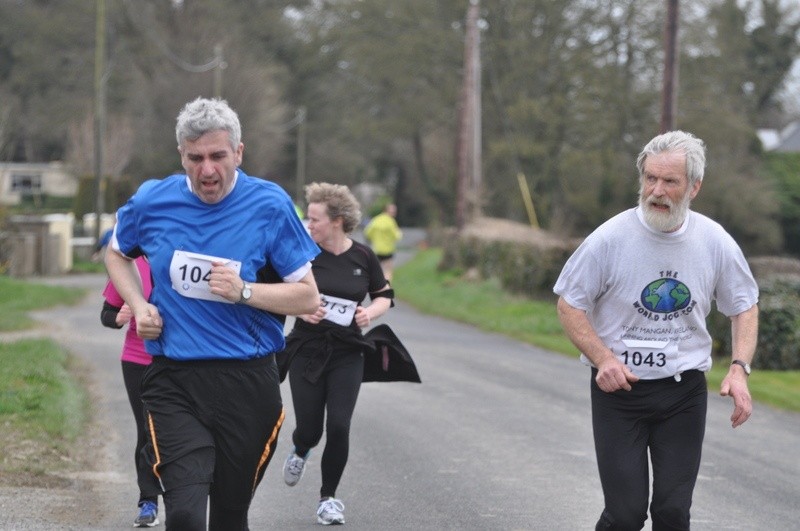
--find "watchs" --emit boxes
[731,359,751,375]
[234,280,253,306]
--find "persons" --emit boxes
[104,96,323,531]
[92,228,114,260]
[363,202,404,285]
[279,182,423,525]
[552,129,760,531]
[100,252,163,528]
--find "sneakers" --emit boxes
[283,449,311,486]
[133,500,159,526]
[316,497,345,525]
